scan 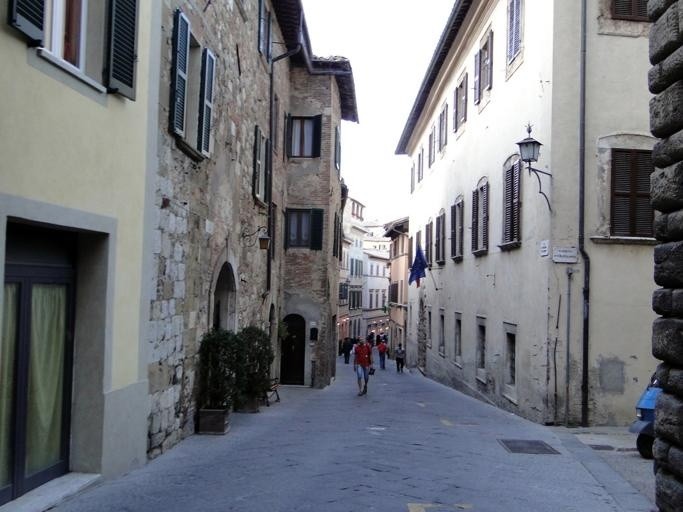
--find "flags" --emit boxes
[409,245,428,285]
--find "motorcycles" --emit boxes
[626,365,661,462]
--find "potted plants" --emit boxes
[191,326,246,436]
[231,323,274,415]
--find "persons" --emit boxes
[342,337,352,364]
[395,344,405,373]
[377,341,388,370]
[354,337,373,396]
[366,333,387,348]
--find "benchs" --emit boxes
[256,378,280,408]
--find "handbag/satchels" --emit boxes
[368,367,375,376]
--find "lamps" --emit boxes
[514,122,553,214]
[240,224,271,251]
